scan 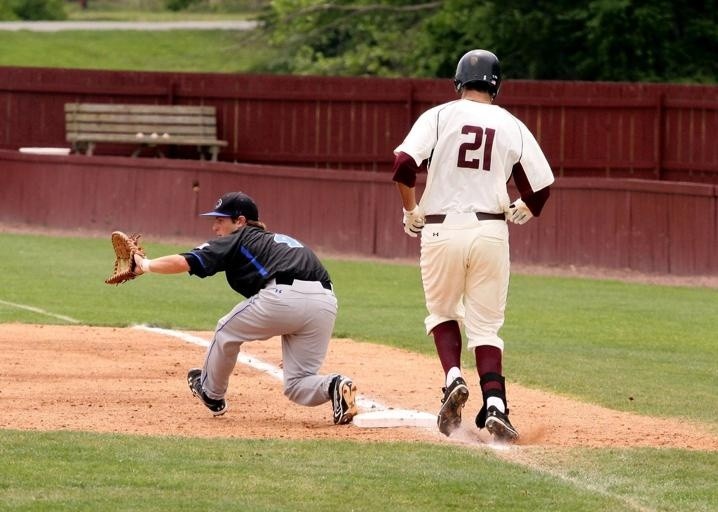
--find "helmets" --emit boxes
[450,48,504,94]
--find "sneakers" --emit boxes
[186,367,229,418]
[481,404,520,446]
[329,374,359,428]
[435,376,469,438]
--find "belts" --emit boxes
[273,276,332,291]
[423,211,507,225]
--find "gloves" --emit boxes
[505,197,534,226]
[400,203,426,238]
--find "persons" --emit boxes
[103,191,358,427]
[390,49,556,442]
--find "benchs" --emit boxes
[64,102,228,163]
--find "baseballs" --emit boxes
[150,131,159,140]
[162,133,170,139]
[135,131,144,139]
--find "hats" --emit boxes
[197,191,261,223]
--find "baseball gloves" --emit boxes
[104,231,147,285]
